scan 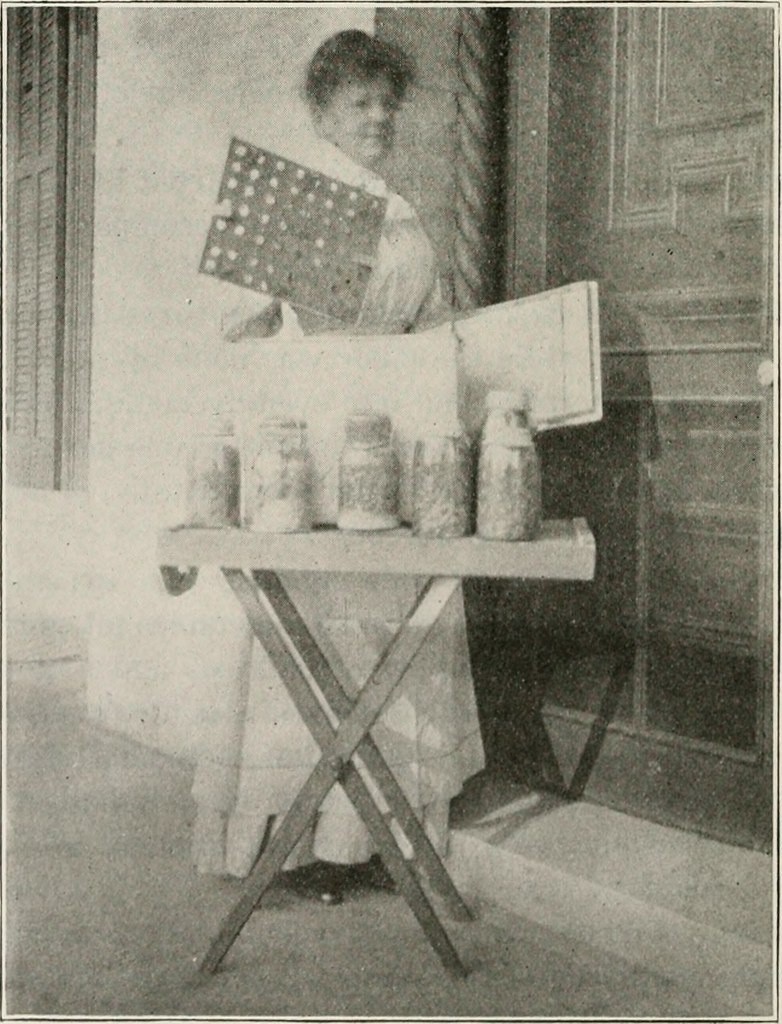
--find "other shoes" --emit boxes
[340,853,396,890]
[294,862,361,905]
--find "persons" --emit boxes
[189,32,487,903]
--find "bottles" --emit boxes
[413,418,470,537]
[477,390,542,539]
[337,412,402,530]
[252,419,319,532]
[201,412,241,526]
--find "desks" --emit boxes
[153,516,594,979]
